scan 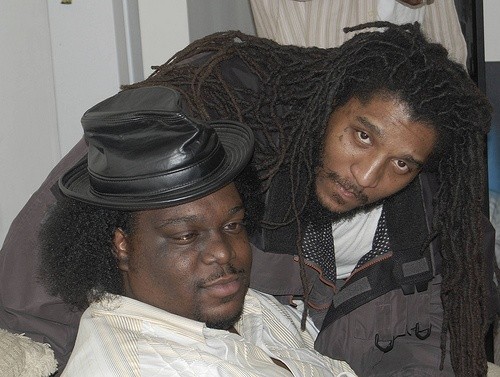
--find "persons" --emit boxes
[37,85,357,376]
[0,21,500,376]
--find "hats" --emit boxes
[58,83,254,210]
